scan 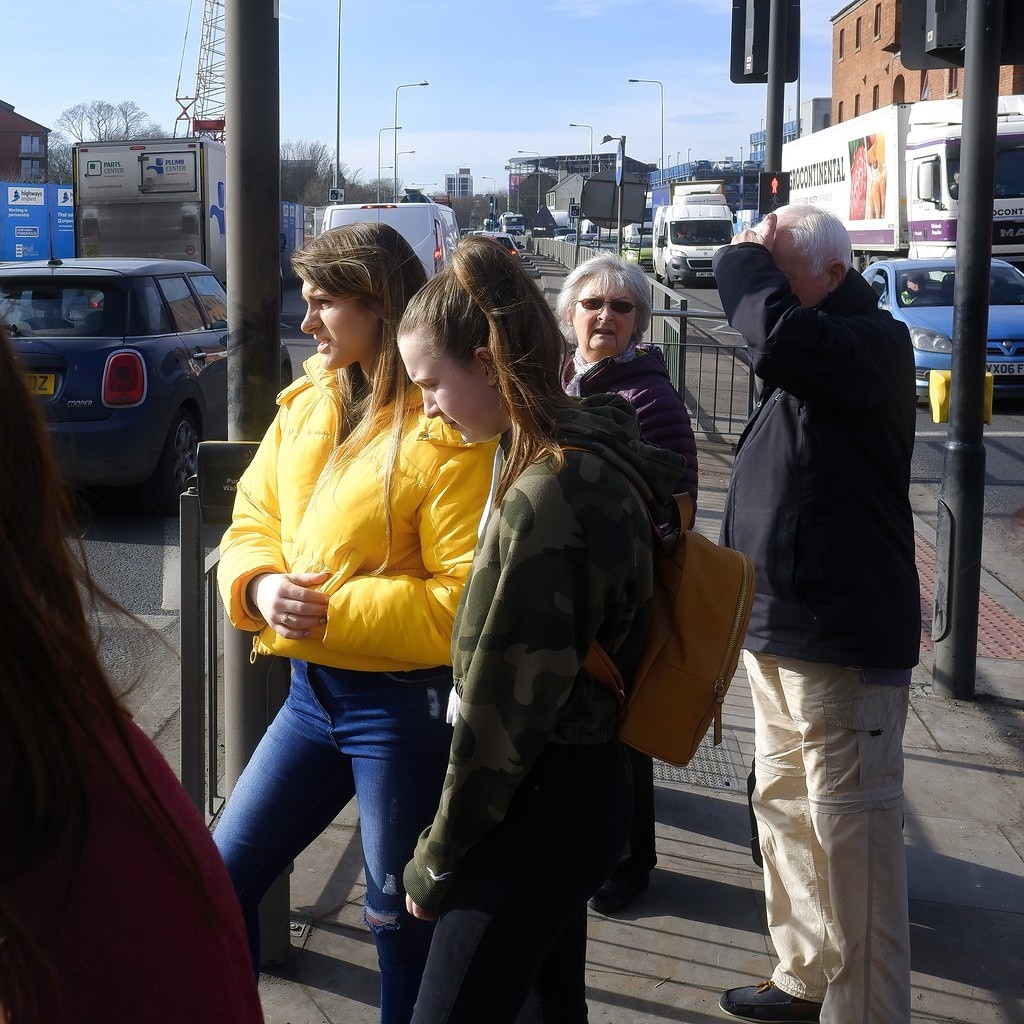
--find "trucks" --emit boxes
[549,210,618,237]
[624,191,652,241]
[651,179,728,226]
[71,140,288,312]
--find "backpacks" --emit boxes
[529,442,756,768]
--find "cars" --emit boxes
[622,236,653,269]
[554,228,596,246]
[859,258,1024,397]
[590,234,619,252]
[460,228,525,259]
[0,255,295,513]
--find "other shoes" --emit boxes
[587,872,649,916]
[718,981,824,1023]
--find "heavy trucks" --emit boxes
[787,94,1024,282]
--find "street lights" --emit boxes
[628,79,664,184]
[599,135,626,254]
[395,82,429,203]
[483,176,495,231]
[504,166,520,212]
[396,151,416,203]
[518,151,541,212]
[377,127,401,202]
[570,124,592,234]
[411,183,437,194]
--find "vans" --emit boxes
[652,205,736,287]
[321,201,460,281]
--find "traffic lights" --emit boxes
[488,196,493,207]
[759,172,790,215]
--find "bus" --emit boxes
[484,212,525,234]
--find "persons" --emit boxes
[900,274,928,305]
[0,329,265,1024]
[394,237,692,1024]
[677,224,691,239]
[556,251,699,915]
[211,224,503,1024]
[711,203,922,1024]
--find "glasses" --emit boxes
[580,298,640,314]
